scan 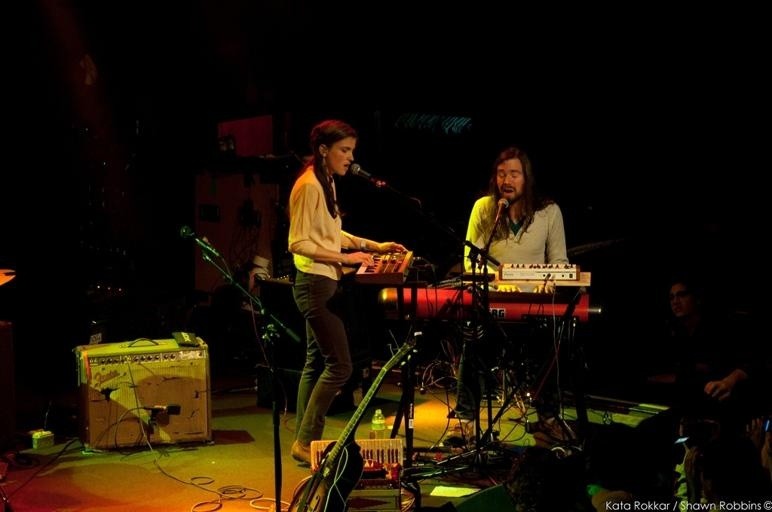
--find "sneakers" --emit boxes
[443,419,474,446]
[538,418,576,441]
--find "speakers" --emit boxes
[257,366,372,416]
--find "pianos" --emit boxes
[311,439,404,478]
[355,247,413,284]
[378,284,591,325]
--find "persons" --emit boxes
[284,119,407,466]
[634,278,766,412]
[744,415,771,479]
[452,146,582,450]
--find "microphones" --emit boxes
[493,198,509,226]
[350,163,371,180]
[180,226,221,259]
[433,268,526,469]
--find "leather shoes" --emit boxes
[291,440,311,464]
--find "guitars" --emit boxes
[289,330,424,512]
